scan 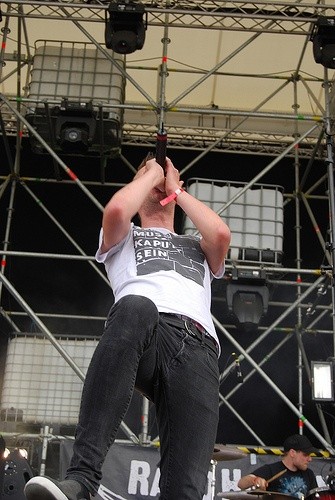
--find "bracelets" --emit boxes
[253,477,259,485]
[160,188,184,206]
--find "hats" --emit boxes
[284,434,318,454]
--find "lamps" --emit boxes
[226,283,270,332]
[310,16,335,69]
[105,2,148,54]
[311,361,334,401]
[54,117,97,151]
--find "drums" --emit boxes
[308,486,335,500]
[262,491,301,500]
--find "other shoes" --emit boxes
[23,475,91,500]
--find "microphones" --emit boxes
[236,359,243,383]
[155,129,167,176]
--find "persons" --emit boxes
[238,435,318,500]
[24,157,231,500]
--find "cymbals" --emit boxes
[210,448,244,461]
[217,490,267,500]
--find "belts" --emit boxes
[160,314,220,356]
